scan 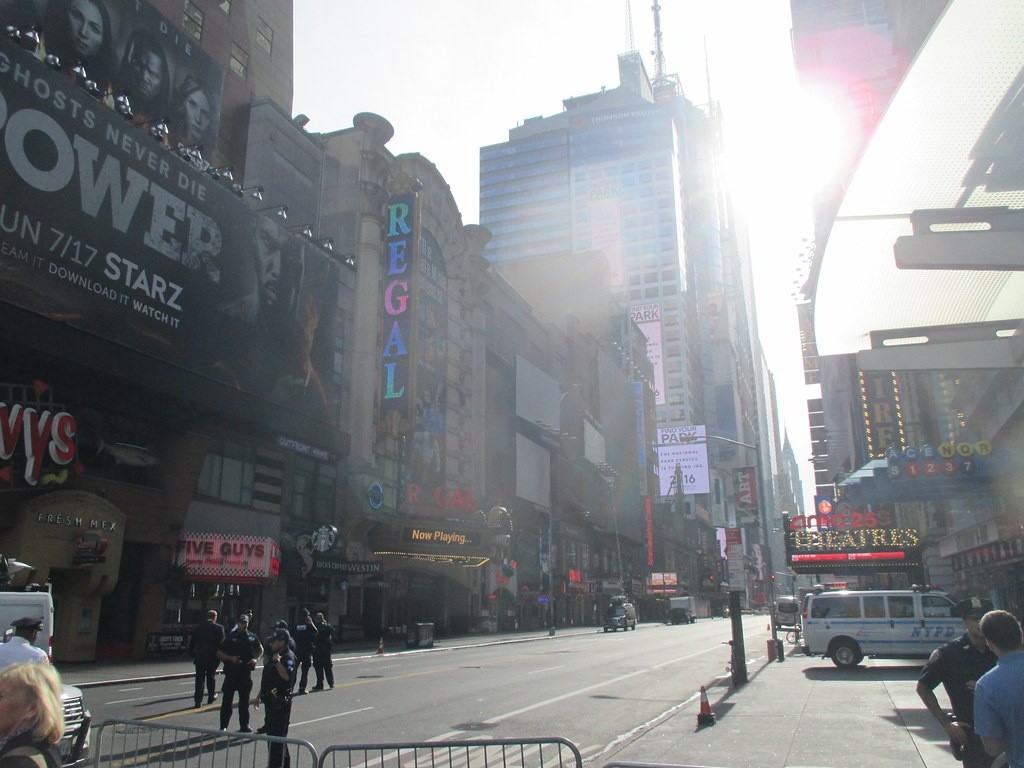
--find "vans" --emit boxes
[773,594,802,631]
[802,582,976,670]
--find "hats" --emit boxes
[955,597,994,619]
[240,609,252,616]
[239,614,250,624]
[11,617,45,632]
[266,628,289,642]
[270,620,288,629]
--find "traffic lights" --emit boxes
[771,573,775,582]
[710,574,713,580]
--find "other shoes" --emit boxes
[240,724,253,731]
[208,693,219,703]
[330,683,334,688]
[298,689,309,695]
[195,700,202,708]
[312,684,323,690]
[216,728,226,737]
[258,726,267,733]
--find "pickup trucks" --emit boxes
[0,568,56,665]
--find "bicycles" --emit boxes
[786,623,803,644]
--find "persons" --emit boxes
[254,629,297,768]
[312,613,336,690]
[119,28,171,116]
[748,545,767,581]
[257,619,295,732]
[915,598,1008,768]
[0,663,66,768]
[189,611,225,708]
[45,0,111,88]
[292,609,318,694]
[216,614,264,732]
[174,76,215,141]
[0,617,49,671]
[221,608,252,690]
[973,609,1024,768]
[252,213,327,406]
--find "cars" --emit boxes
[0,628,92,768]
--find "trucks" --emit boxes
[670,596,697,625]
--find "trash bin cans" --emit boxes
[406,623,435,650]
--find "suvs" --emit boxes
[603,603,638,633]
